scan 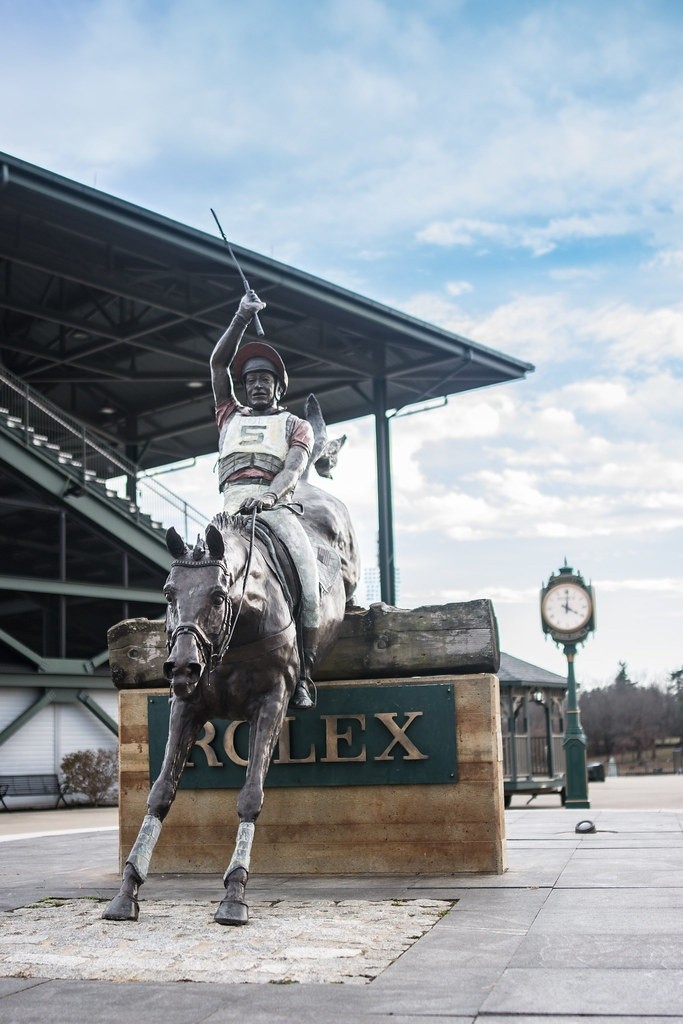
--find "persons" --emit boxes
[210,288,321,709]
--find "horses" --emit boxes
[100,391,363,928]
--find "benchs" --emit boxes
[0,775,69,812]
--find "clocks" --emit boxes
[542,583,593,633]
